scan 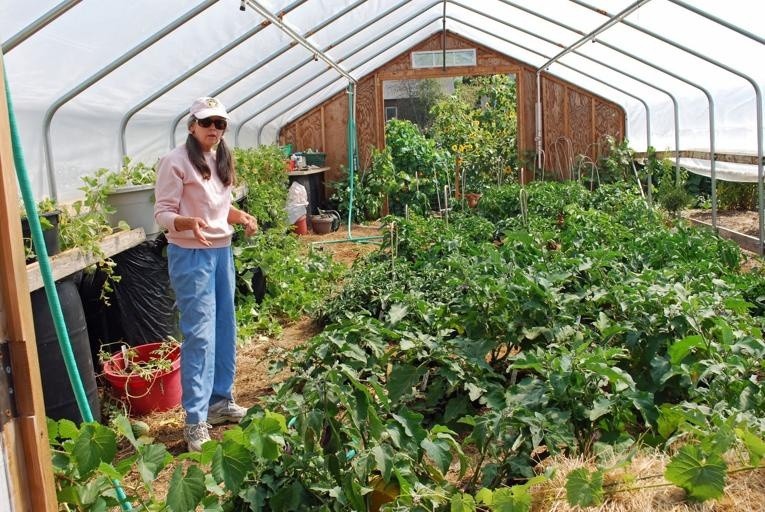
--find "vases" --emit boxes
[310,214,318,233]
[315,218,331,234]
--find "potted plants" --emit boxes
[92,331,186,416]
[54,153,163,308]
[14,194,62,269]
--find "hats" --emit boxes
[190,96,230,121]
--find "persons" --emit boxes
[153,96,258,456]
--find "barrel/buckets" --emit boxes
[28,279,102,443]
[102,342,182,416]
[95,182,161,235]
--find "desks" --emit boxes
[284,162,332,238]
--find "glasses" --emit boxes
[192,117,227,130]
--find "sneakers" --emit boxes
[182,420,214,459]
[206,397,252,427]
